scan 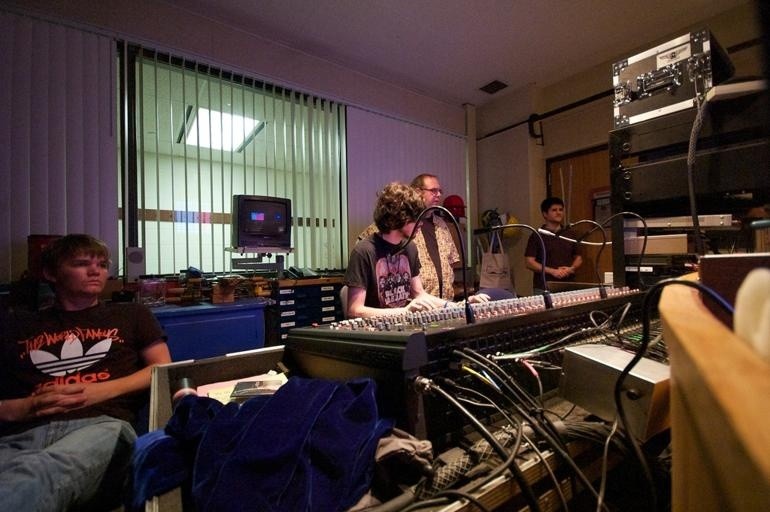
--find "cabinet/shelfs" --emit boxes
[265,277,345,349]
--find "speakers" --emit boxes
[126,247,144,283]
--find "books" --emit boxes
[207,372,288,406]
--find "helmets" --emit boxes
[502,215,520,239]
[443,195,467,219]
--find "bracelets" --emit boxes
[444,301,450,308]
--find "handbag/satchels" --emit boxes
[480,253,512,290]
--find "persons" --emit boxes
[356,173,460,301]
[523,196,583,295]
[343,181,491,320]
[0,233,175,512]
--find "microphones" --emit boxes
[555,220,608,299]
[538,228,612,245]
[390,206,475,324]
[474,224,554,309]
[576,212,648,292]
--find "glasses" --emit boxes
[421,188,442,195]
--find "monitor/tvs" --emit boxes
[231,195,291,249]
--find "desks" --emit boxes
[151,297,276,364]
